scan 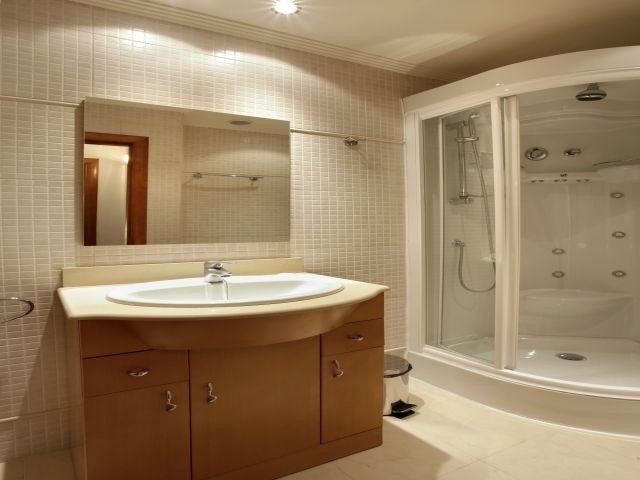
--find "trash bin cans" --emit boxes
[382,354,417,416]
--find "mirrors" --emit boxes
[83,95,291,246]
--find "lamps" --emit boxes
[575,81,606,102]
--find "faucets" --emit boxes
[203,260,238,283]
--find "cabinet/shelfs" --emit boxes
[67,316,191,480]
[320,291,385,466]
[190,334,320,479]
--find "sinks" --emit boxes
[105,273,346,307]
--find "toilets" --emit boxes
[517,240,633,335]
